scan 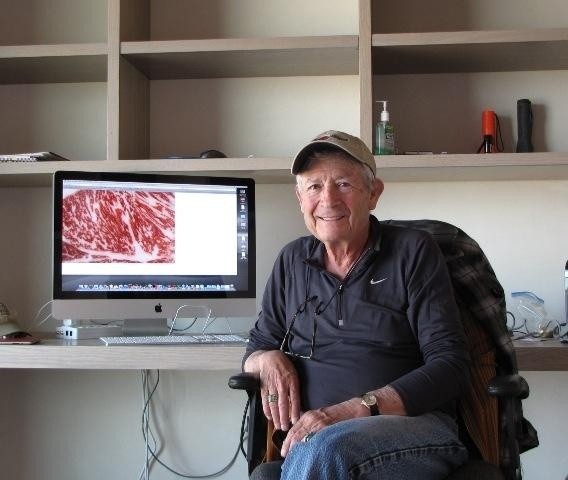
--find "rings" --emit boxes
[268,393,277,404]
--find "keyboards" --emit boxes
[96,334,248,347]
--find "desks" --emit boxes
[0,328,568,372]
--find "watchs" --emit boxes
[360,392,381,415]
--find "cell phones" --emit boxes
[0,337,40,345]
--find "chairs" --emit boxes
[228,219,528,480]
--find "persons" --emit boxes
[241,130,473,480]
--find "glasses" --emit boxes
[279,295,325,362]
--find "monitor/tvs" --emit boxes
[52,170,257,336]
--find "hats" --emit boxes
[291,130,377,176]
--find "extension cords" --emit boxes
[56,326,123,339]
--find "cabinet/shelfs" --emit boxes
[1,0,567,186]
